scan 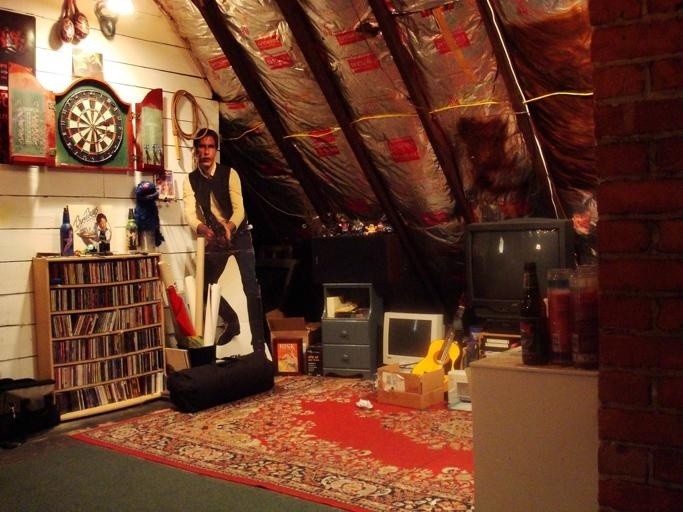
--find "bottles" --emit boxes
[60,204,75,257]
[518,257,598,372]
[125,207,139,253]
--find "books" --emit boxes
[50,256,165,416]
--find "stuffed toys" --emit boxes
[134,180,166,246]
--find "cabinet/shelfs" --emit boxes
[463,349,602,512]
[32,251,170,427]
[323,278,382,379]
[478,331,524,362]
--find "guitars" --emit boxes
[411,305,465,393]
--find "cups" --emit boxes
[139,229,155,254]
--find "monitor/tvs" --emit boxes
[465,219,571,318]
[382,312,445,365]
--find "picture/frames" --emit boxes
[271,337,305,376]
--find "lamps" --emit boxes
[95,2,134,37]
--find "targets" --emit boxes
[59,90,124,166]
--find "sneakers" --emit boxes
[215,319,243,347]
[252,343,268,361]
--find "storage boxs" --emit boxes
[263,309,323,357]
[375,363,445,410]
[301,343,324,378]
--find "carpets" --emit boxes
[66,375,477,512]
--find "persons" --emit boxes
[182,129,267,353]
[89,214,110,244]
